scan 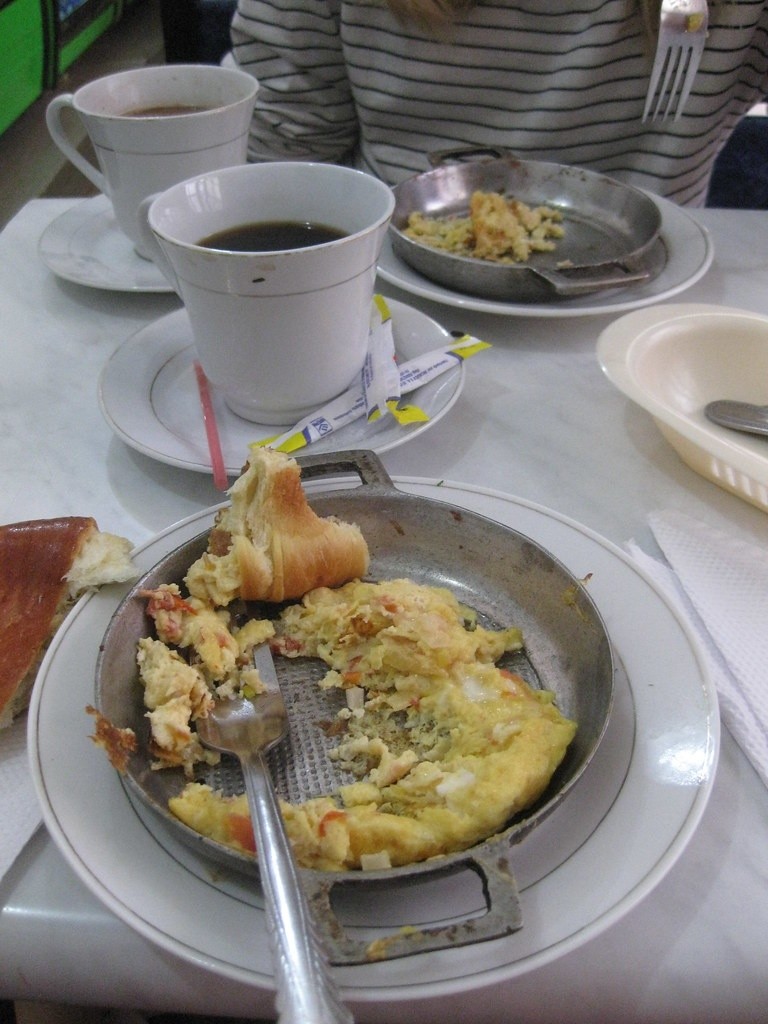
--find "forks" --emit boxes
[642,0,708,124]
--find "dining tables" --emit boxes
[0,196,767,1023]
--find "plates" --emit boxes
[36,191,181,293]
[377,184,713,320]
[29,471,724,1001]
[96,293,468,478]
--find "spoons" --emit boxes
[187,597,357,1024]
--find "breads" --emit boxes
[0,515,139,732]
[183,448,370,606]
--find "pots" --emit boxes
[91,450,618,972]
[386,144,663,305]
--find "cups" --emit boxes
[135,160,397,428]
[45,63,259,266]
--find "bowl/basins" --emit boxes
[594,303,768,515]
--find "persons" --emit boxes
[219,0,768,208]
